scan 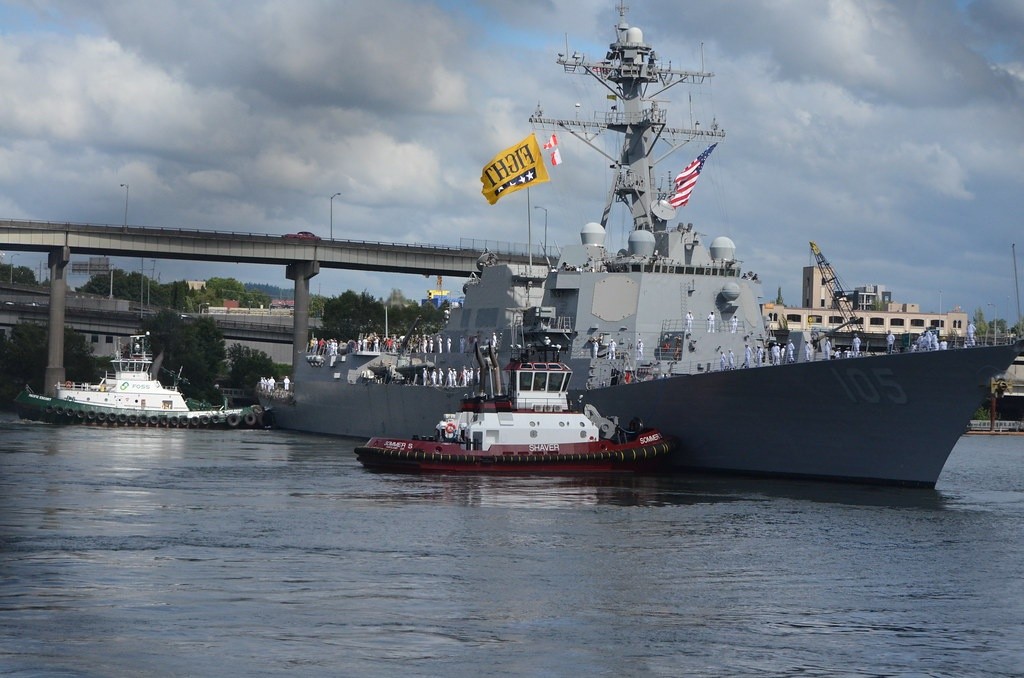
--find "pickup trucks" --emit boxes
[282,232,322,241]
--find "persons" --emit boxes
[743,273,758,280]
[552,263,608,273]
[965,319,977,347]
[592,340,599,358]
[707,311,716,332]
[260,375,290,395]
[686,311,694,332]
[911,327,948,351]
[719,349,735,371]
[544,336,551,346]
[730,316,737,333]
[743,344,752,369]
[635,340,643,359]
[755,334,860,367]
[886,330,895,355]
[607,339,617,360]
[309,332,498,441]
[134,342,141,354]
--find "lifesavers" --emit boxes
[149,416,158,425]
[227,414,240,427]
[201,416,209,425]
[348,340,356,348]
[446,422,456,434]
[212,415,220,424]
[108,413,117,422]
[118,414,127,423]
[180,417,190,426]
[139,415,148,424]
[170,416,179,426]
[191,416,199,425]
[66,381,72,388]
[45,405,96,420]
[629,416,644,437]
[160,416,168,426]
[98,412,107,421]
[129,414,138,424]
[243,413,256,426]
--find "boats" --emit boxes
[353,325,671,473]
[13,332,274,429]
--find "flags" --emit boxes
[669,143,718,209]
[479,133,562,205]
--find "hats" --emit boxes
[545,337,549,339]
[720,351,724,352]
[805,340,808,342]
[734,315,737,316]
[611,339,613,341]
[745,344,748,346]
[757,345,761,348]
[789,339,792,342]
[728,349,732,351]
[688,311,692,312]
[774,342,777,344]
[639,339,641,341]
[826,337,830,339]
[711,311,714,312]
[423,366,480,370]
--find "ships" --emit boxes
[259,1,1024,493]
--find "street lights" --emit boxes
[11,254,21,284]
[330,193,341,241]
[988,304,996,346]
[534,206,547,256]
[939,290,942,338]
[120,184,129,225]
[380,301,388,338]
[139,269,150,305]
[199,303,210,315]
